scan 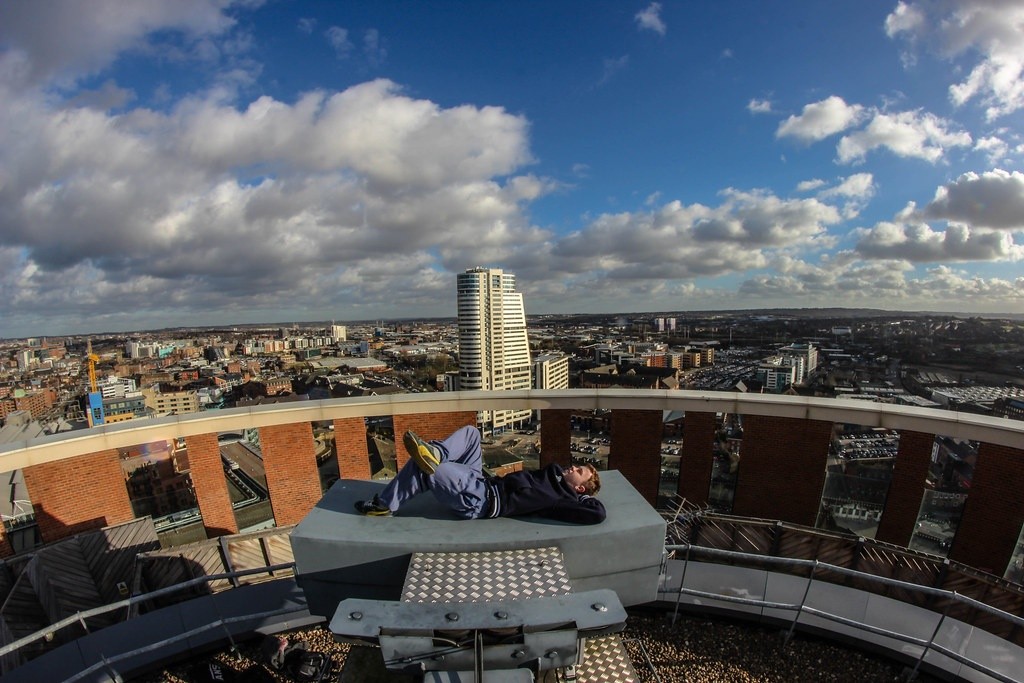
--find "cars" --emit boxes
[833,429,901,459]
[660,437,684,481]
[570,437,611,466]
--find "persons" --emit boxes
[354,425,606,525]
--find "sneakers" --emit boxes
[403,430,440,475]
[353,493,399,517]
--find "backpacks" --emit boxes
[283,648,338,683]
